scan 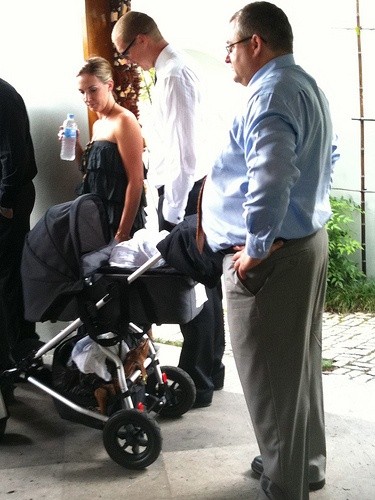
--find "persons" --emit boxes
[196,1,342,500]
[58,11,225,408]
[0,78,41,372]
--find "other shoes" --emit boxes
[251,455,325,492]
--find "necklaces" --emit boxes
[99,102,116,128]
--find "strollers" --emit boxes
[1,191,223,471]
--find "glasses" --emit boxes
[226,35,264,53]
[118,33,146,59]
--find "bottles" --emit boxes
[60,114,77,161]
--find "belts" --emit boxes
[158,185,164,197]
[216,245,238,256]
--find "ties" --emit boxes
[196,174,207,254]
